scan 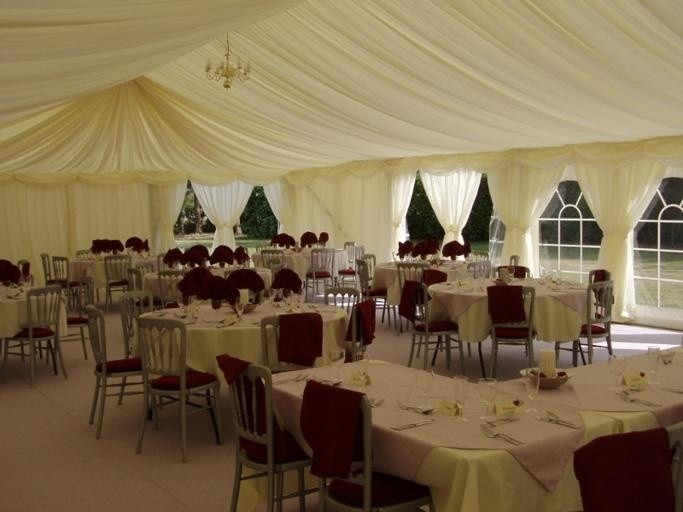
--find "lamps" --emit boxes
[205,32,251,89]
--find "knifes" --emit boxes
[392,419,432,431]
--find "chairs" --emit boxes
[1,226,388,377]
[553,279,616,367]
[397,261,434,336]
[300,379,432,512]
[570,426,678,510]
[486,286,538,376]
[510,254,521,265]
[402,279,465,371]
[133,316,224,463]
[590,268,612,285]
[497,266,531,280]
[473,249,492,261]
[79,304,143,439]
[466,260,492,281]
[213,355,318,511]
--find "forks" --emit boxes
[479,424,531,451]
[272,372,308,386]
[614,392,659,408]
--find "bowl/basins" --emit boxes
[519,367,570,389]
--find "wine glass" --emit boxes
[453,374,469,423]
[524,364,541,414]
[475,376,498,420]
[606,354,628,393]
[0,272,32,301]
[644,345,661,387]
[388,249,516,278]
[326,346,349,388]
[354,350,371,395]
[171,286,277,325]
[413,369,435,411]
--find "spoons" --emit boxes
[547,410,586,428]
